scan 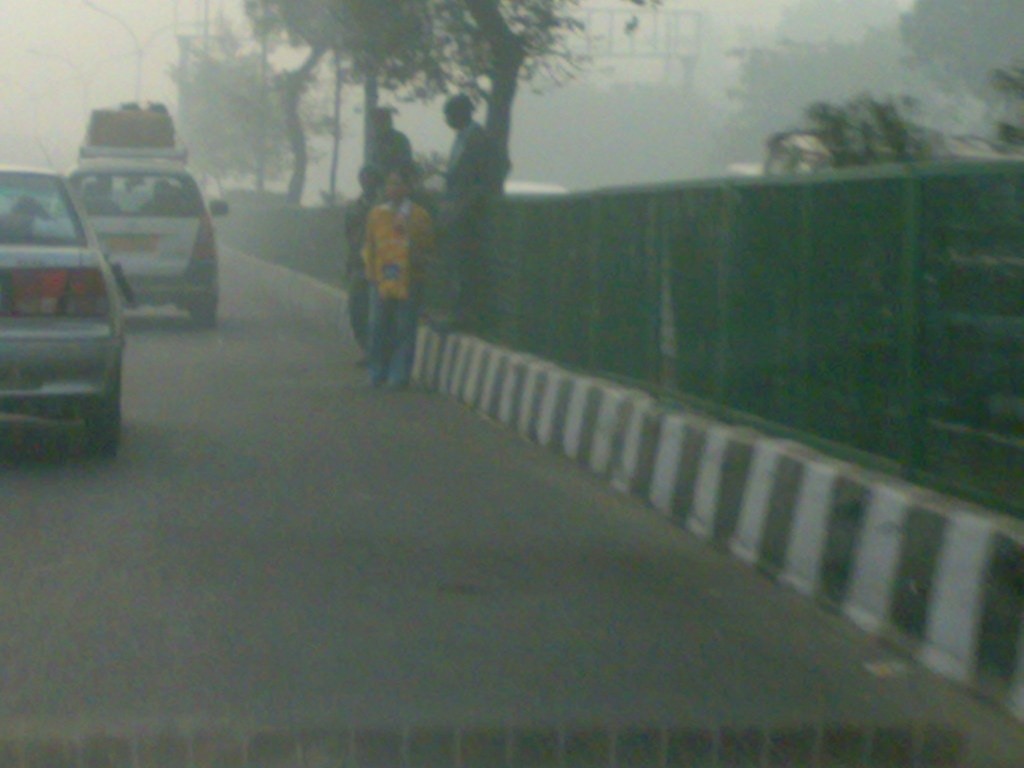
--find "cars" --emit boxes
[0,166,130,456]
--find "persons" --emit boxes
[343,94,489,371]
[361,166,435,395]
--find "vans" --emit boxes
[60,160,232,330]
